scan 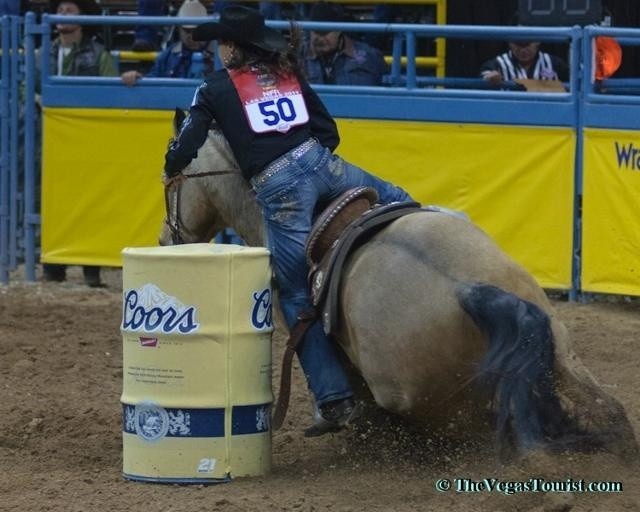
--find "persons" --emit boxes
[161,2,414,437]
[477,21,564,94]
[17,0,115,289]
[120,0,431,87]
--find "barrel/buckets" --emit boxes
[121,243,274,484]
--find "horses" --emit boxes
[159,106,639,463]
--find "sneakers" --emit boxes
[305,399,352,437]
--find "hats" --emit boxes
[168,0,206,44]
[280,3,374,22]
[193,5,292,54]
